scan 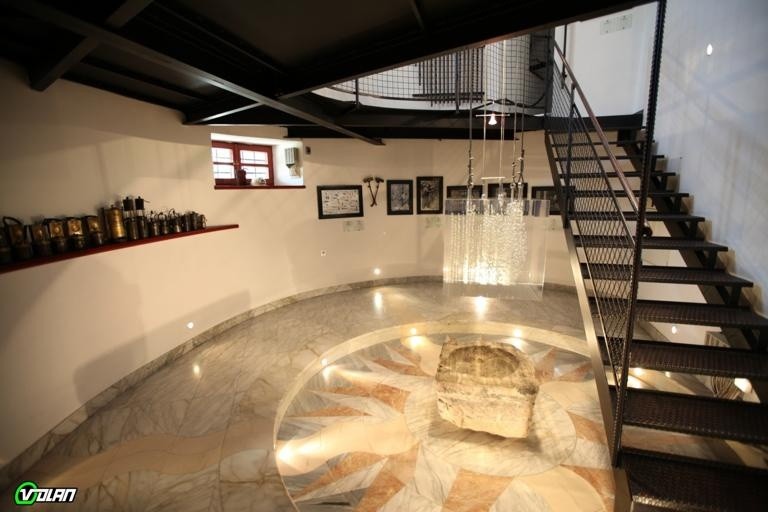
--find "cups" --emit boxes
[234,170,248,185]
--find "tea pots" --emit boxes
[249,176,267,187]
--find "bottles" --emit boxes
[0,196,208,264]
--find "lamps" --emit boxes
[488,113,497,125]
[443,42,550,301]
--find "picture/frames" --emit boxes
[387,180,413,215]
[445,186,484,214]
[416,176,443,214]
[317,185,363,219]
[532,186,575,215]
[488,184,528,215]
